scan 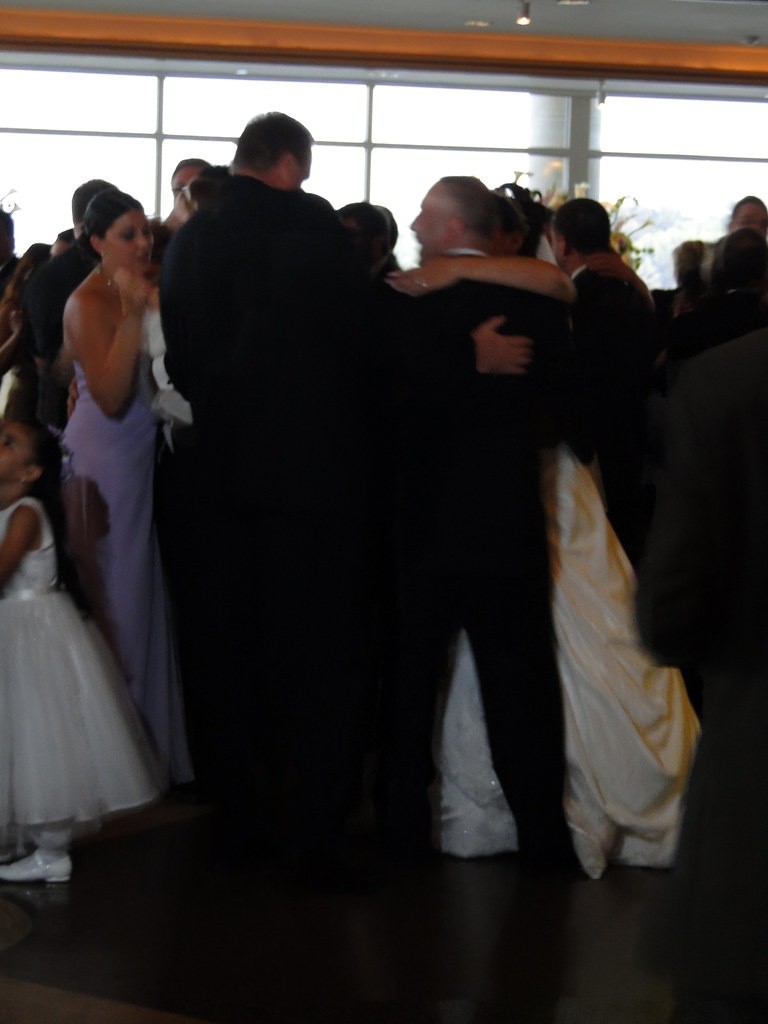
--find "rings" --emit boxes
[412,278,432,290]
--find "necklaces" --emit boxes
[95,265,118,293]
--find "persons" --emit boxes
[0,111,768,888]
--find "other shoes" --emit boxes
[0,842,30,863]
[0,851,71,883]
